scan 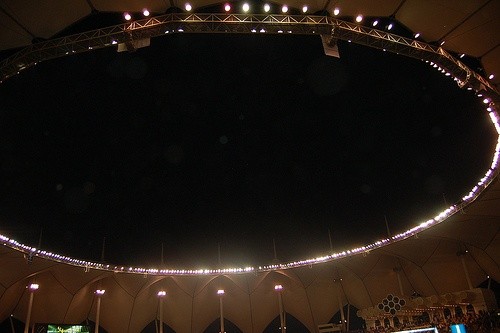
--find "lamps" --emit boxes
[274,284,283,290]
[157,291,167,296]
[217,289,224,294]
[95,289,105,295]
[29,282,40,290]
